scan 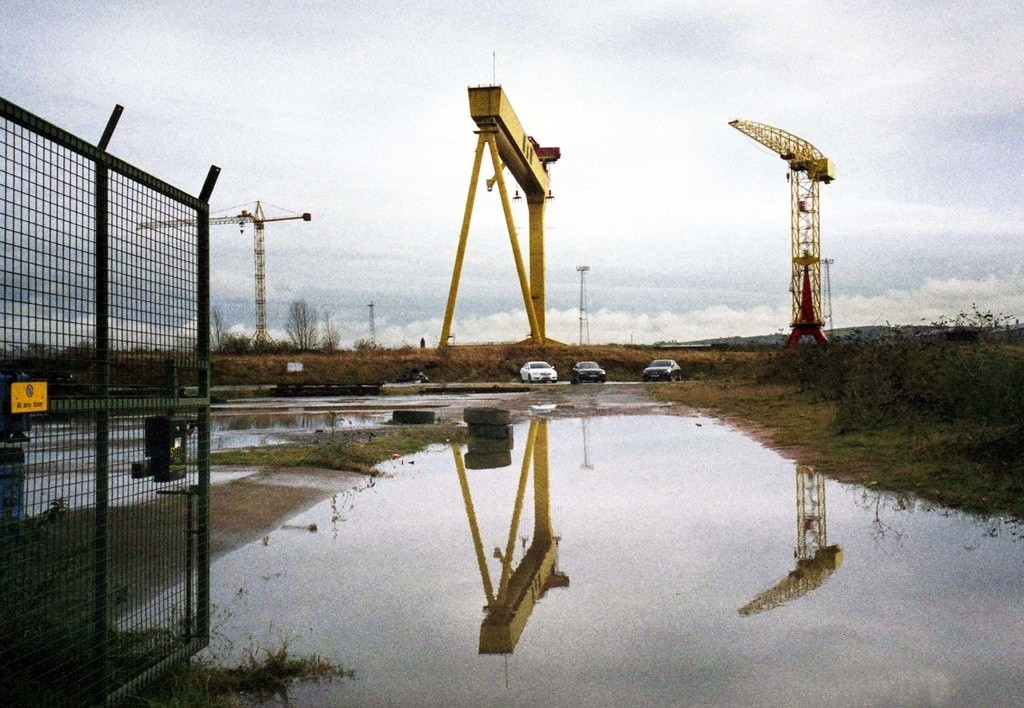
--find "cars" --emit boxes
[521,360,558,384]
[642,358,683,381]
[572,360,607,384]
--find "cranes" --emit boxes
[728,116,837,351]
[135,199,311,345]
[735,458,844,621]
